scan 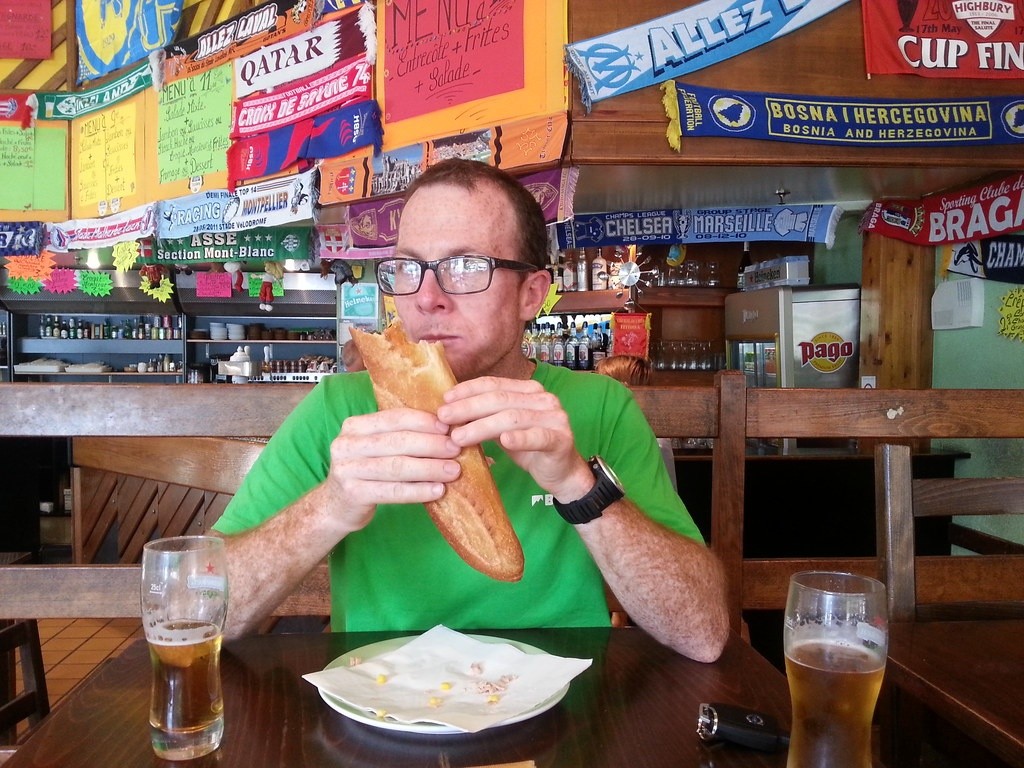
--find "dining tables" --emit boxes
[0,629,795,768]
[881,617,1023,761]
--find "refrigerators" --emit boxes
[725,283,861,456]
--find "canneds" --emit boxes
[151,314,181,340]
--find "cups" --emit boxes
[783,568,889,768]
[140,534,230,762]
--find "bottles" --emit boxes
[577,247,589,292]
[592,247,607,290]
[563,252,578,292]
[521,321,612,370]
[156,353,183,372]
[39,314,152,340]
[736,241,754,292]
[553,250,565,293]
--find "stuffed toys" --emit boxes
[138,263,167,289]
[172,263,192,275]
[224,261,244,293]
[251,261,283,312]
[321,259,357,286]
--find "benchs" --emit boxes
[0,370,1023,627]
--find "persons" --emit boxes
[166,157,731,662]
[597,356,678,626]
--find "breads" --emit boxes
[346,317,527,583]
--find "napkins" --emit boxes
[302,624,594,735]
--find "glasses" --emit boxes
[374,255,538,296]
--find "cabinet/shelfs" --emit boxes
[176,268,340,384]
[521,240,814,385]
[0,268,184,384]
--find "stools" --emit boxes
[0,549,50,745]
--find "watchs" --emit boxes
[553,454,625,524]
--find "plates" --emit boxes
[318,633,571,735]
[189,322,300,340]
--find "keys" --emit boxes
[699,703,790,750]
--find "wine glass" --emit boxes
[657,259,719,287]
[650,338,726,371]
